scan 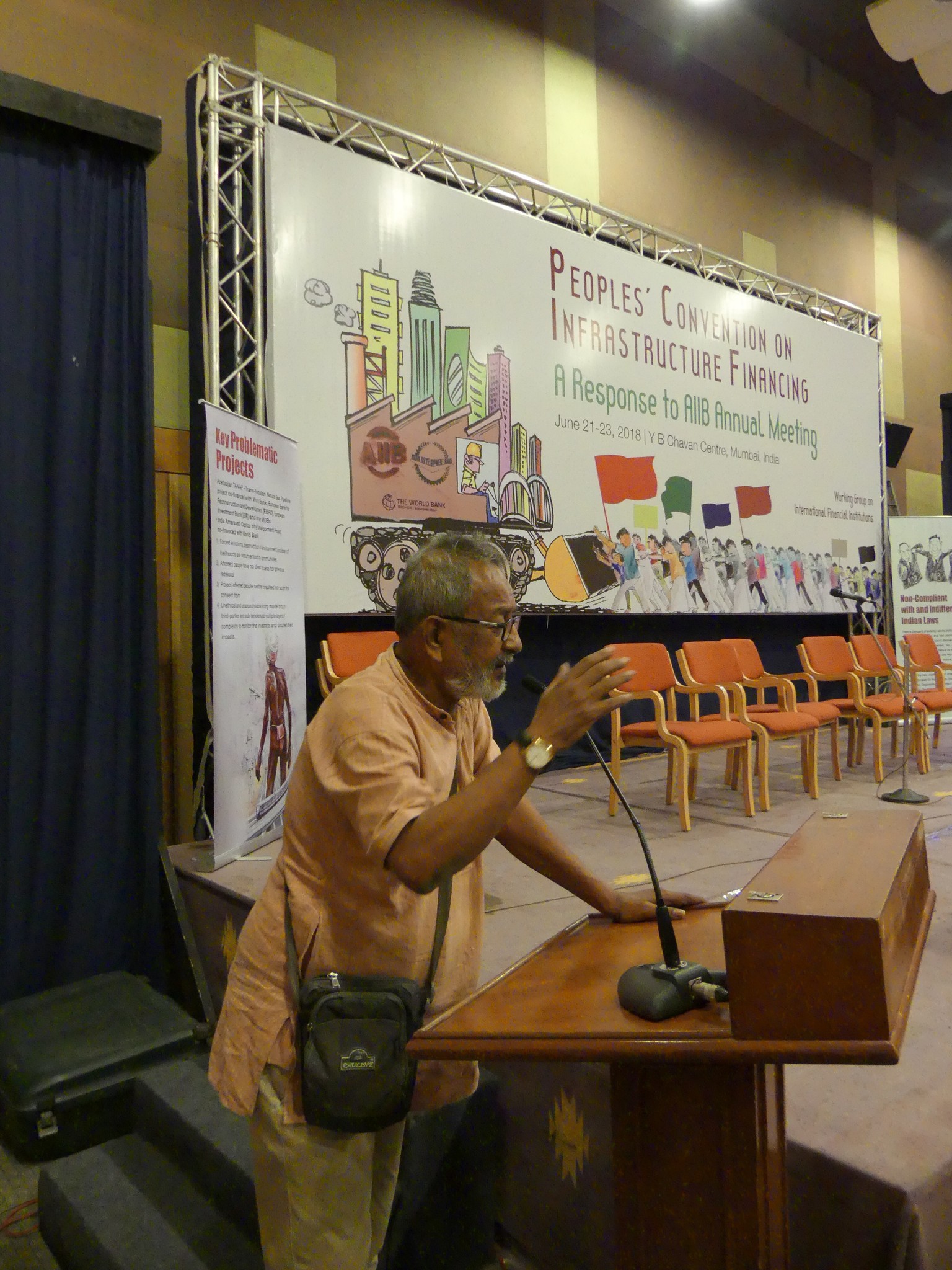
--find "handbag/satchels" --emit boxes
[294,973,426,1133]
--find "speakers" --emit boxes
[886,422,914,468]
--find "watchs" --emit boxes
[513,728,557,775]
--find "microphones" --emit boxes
[829,589,877,604]
[521,675,712,1021]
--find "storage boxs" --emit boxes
[0,972,202,1165]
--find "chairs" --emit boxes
[602,642,755,831]
[723,638,842,792]
[797,634,952,783]
[316,631,401,699]
[675,641,821,810]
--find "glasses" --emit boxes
[439,614,522,641]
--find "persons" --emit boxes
[207,527,706,1270]
